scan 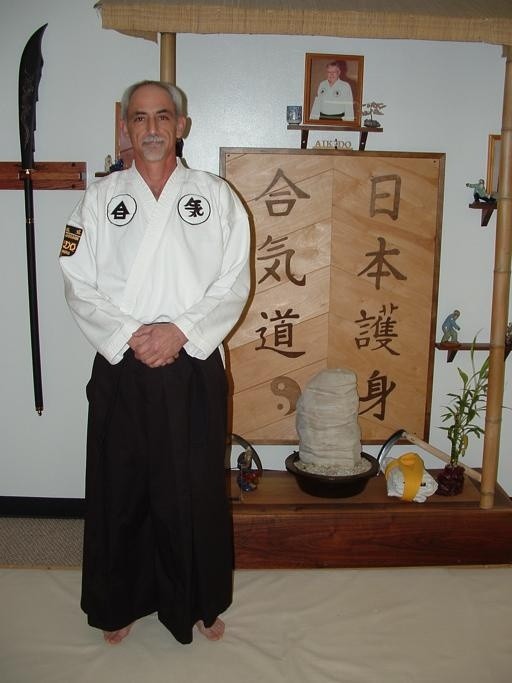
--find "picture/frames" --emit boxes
[304,52,365,127]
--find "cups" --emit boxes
[286,105,302,126]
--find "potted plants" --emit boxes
[435,328,510,496]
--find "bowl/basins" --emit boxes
[285,450,381,499]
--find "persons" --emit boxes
[110,156,125,171]
[58,80,256,646]
[439,308,462,344]
[310,60,355,121]
[102,154,113,170]
[465,178,494,203]
[235,444,262,494]
